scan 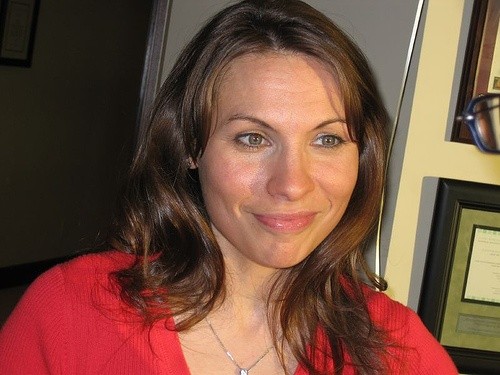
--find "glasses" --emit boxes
[457,93,500,153]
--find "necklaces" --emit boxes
[188,277,287,375]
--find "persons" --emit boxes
[0,1,458,375]
